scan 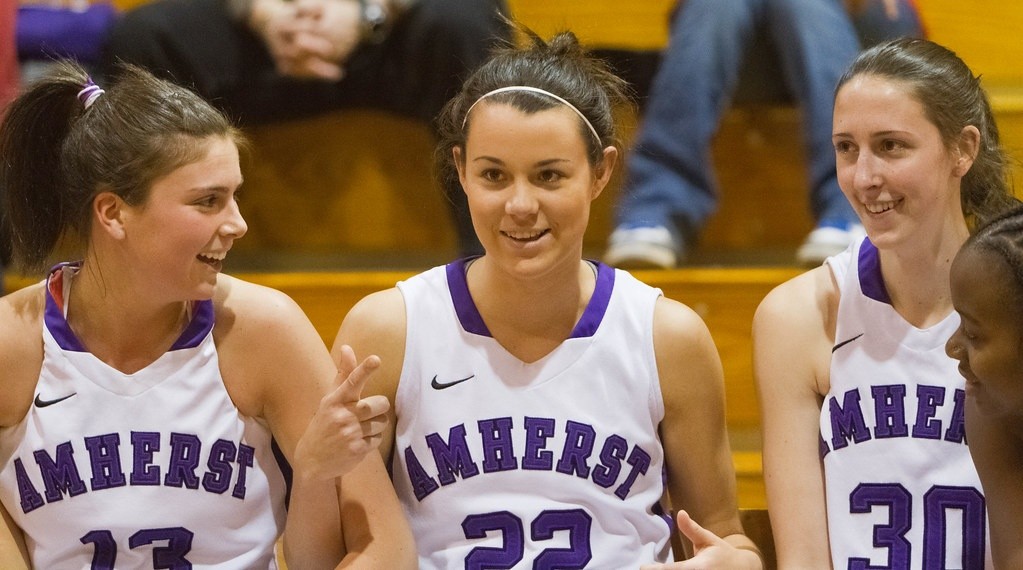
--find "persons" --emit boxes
[604,0,862,268]
[282,13,766,570]
[96,1,516,261]
[0,60,422,569]
[750,34,1014,570]
[944,204,1023,570]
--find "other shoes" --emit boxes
[795,227,856,266]
[601,226,676,270]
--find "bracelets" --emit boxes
[362,2,389,42]
[736,546,765,570]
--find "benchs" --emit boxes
[0,0,1023,570]
